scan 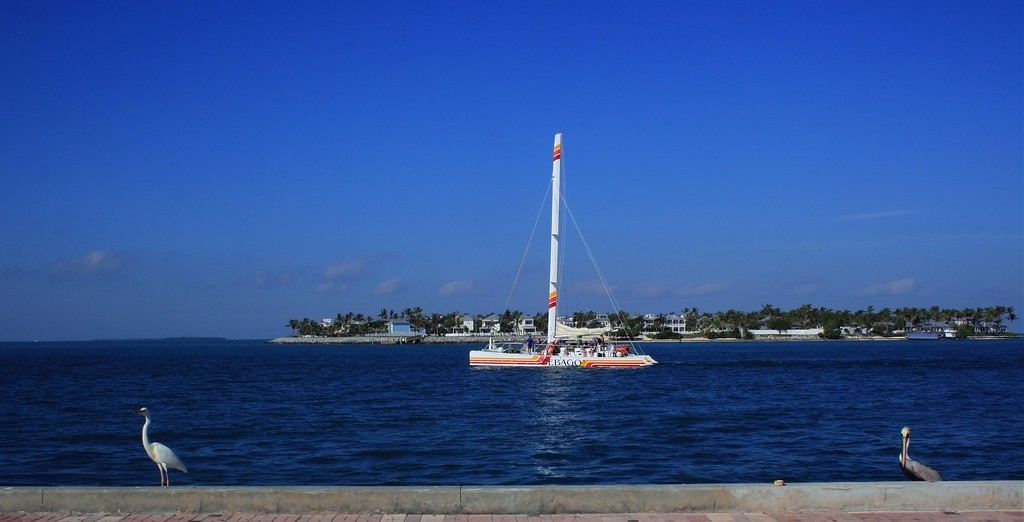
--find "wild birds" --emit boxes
[897,426,942,482]
[131,407,189,486]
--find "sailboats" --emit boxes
[468,131,661,369]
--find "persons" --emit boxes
[537,339,541,350]
[525,336,533,352]
[545,335,605,357]
